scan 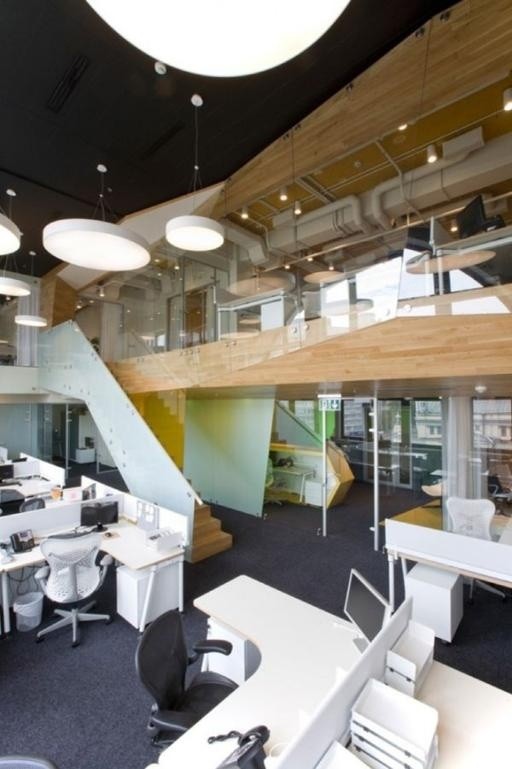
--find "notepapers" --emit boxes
[137,502,141,510]
[145,503,150,511]
[149,507,154,514]
[145,514,152,522]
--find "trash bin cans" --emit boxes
[12,591,44,632]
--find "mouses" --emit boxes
[105,532,111,537]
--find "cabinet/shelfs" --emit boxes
[263,442,355,509]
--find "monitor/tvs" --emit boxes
[0,465,14,486]
[13,458,27,463]
[81,501,119,532]
[457,195,487,240]
[82,483,96,500]
[343,567,391,654]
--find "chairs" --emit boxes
[445,498,506,602]
[134,609,239,749]
[34,532,113,649]
[13,754,58,767]
[454,195,504,237]
[18,498,47,512]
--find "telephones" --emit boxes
[208,725,270,768]
[9,528,35,553]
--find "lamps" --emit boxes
[14,315,48,327]
[164,100,225,252]
[294,200,301,215]
[279,187,288,201]
[0,188,32,297]
[449,218,458,232]
[240,207,249,220]
[42,164,152,272]
[99,288,105,297]
[503,87,512,112]
[0,188,23,256]
[426,146,437,165]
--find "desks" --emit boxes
[156,573,512,769]
[0,475,190,636]
[0,451,66,502]
[382,497,512,613]
[428,216,512,296]
[216,287,298,341]
